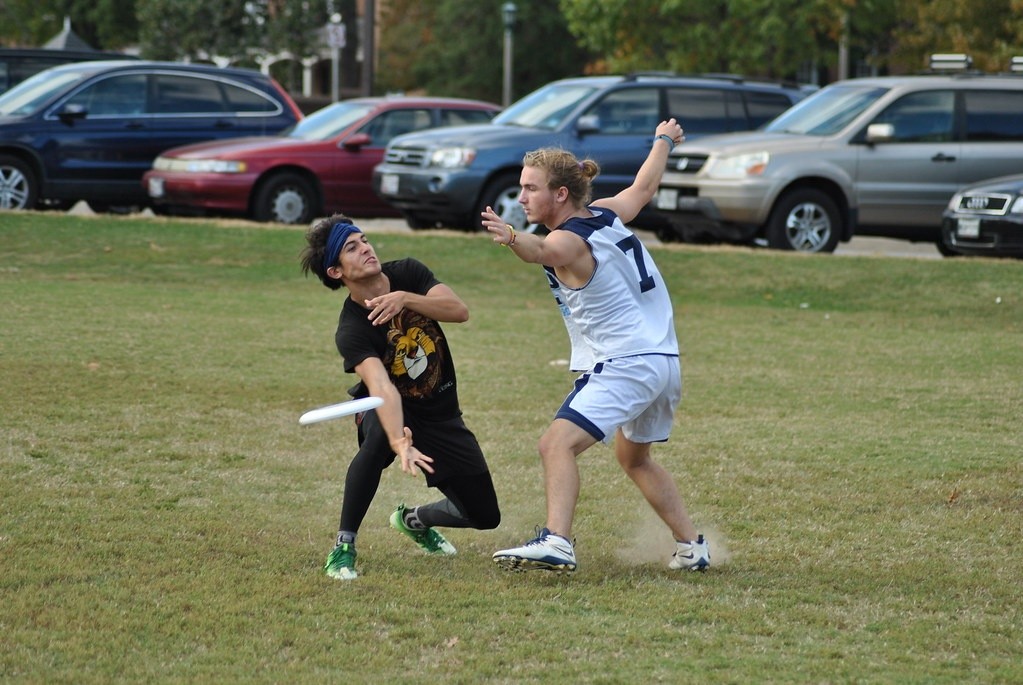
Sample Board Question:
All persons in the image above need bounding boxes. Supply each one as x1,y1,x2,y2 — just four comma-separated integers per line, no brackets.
481,118,711,574
303,216,500,580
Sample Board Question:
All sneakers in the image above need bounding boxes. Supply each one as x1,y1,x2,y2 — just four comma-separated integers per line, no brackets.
492,525,578,576
669,534,712,573
389,503,457,557
323,544,357,580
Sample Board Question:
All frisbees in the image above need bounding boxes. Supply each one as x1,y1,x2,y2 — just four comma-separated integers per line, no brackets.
297,395,385,426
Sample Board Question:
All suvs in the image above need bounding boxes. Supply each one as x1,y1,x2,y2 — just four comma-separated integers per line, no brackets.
0,49,153,110
641,70,1023,259
0,60,306,212
372,71,823,235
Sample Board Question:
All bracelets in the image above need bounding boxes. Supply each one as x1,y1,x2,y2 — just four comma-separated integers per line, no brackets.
500,224,516,247
653,134,681,152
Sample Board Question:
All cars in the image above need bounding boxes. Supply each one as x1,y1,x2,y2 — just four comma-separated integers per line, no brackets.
939,172,1023,260
142,92,508,226
